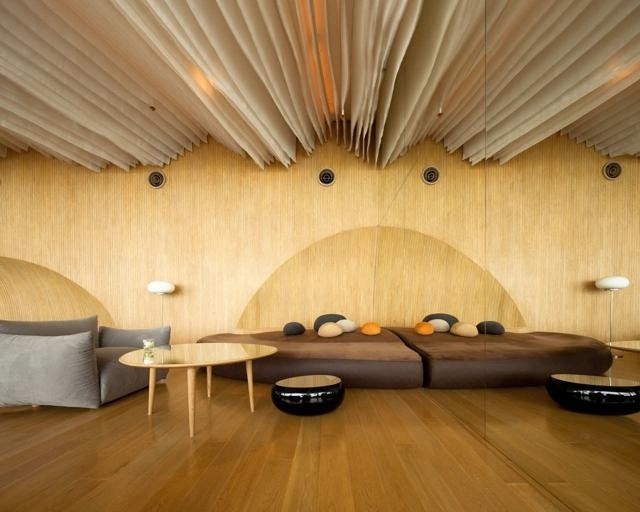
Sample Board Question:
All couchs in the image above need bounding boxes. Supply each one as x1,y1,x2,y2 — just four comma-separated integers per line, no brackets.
0,315,171,408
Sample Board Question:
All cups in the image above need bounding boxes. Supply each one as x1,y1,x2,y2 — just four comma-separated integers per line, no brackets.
143,352,154,364
143,339,155,352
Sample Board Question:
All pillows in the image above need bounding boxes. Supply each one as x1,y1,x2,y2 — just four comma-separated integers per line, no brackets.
283,314,381,336
415,313,505,337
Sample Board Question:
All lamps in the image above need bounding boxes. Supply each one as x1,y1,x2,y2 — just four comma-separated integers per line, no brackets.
148,281,175,328
595,276,629,357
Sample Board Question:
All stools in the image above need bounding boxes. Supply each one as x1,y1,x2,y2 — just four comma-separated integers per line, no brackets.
545,370,640,416
271,375,345,416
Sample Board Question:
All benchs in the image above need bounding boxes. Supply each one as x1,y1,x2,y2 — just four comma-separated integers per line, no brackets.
386,327,613,389
196,325,424,390
